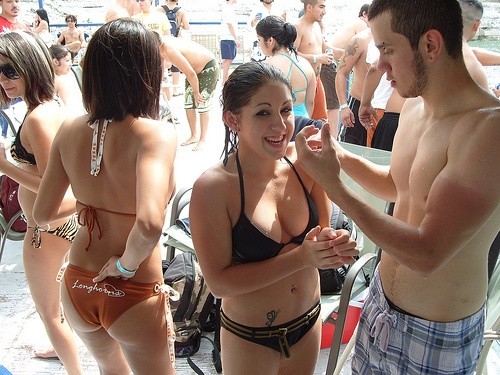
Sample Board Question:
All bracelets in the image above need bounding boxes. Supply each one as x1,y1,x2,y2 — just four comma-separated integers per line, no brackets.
116,259,136,275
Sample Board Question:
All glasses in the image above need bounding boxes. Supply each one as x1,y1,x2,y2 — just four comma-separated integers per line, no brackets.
0,63,21,80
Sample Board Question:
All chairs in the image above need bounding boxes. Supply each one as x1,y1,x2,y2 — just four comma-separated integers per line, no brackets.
0,207,28,263
326,200,500,375
166,185,357,263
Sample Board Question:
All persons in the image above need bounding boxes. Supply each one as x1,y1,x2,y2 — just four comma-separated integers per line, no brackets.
0,30,84,375
293,0,500,152
295,0,500,374
189,62,359,375
256,16,317,118
106,0,189,103
32,19,176,375
0,0,86,116
152,30,219,151
248,0,280,63
219,0,240,85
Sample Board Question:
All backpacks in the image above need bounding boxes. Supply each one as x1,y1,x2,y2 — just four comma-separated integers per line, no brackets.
161,5,182,37
162,253,217,359
0,175,28,232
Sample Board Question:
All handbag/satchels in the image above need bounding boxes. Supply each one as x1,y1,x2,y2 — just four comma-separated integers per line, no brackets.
318,268,342,294
